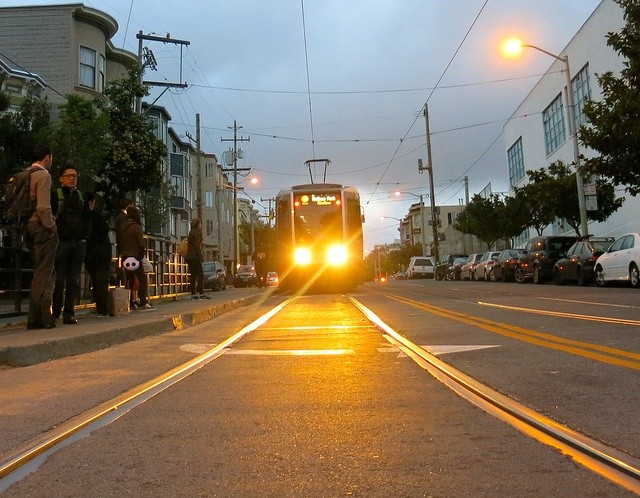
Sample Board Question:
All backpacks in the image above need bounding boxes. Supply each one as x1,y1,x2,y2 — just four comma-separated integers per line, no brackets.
4,166,44,234
56,187,89,241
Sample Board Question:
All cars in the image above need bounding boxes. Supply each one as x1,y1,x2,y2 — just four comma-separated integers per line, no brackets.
593,233,640,287
553,241,615,287
266,272,278,285
449,257,467,280
490,249,523,281
462,253,483,279
395,273,404,279
200,261,226,291
473,252,502,281
234,265,260,288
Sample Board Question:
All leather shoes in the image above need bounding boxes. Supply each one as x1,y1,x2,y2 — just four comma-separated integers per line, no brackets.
63,318,77,324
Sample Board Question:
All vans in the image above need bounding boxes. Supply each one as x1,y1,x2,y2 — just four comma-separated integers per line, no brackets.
515,236,577,284
436,254,469,281
407,257,434,279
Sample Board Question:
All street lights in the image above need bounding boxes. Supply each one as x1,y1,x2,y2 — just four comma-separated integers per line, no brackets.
381,216,406,275
395,191,427,257
236,177,258,186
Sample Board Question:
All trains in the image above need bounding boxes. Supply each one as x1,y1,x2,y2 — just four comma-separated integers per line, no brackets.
277,184,366,293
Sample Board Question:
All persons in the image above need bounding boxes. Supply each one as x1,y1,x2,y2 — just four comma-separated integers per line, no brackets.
176,219,211,301
5,147,60,330
119,207,154,312
83,192,116,318
251,242,271,288
114,200,134,289
50,164,90,324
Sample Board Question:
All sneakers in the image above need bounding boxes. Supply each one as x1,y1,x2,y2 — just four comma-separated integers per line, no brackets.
200,294,211,299
131,302,140,309
191,295,203,301
139,303,157,311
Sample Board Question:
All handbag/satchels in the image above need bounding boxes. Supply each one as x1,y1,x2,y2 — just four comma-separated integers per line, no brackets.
177,237,189,257
